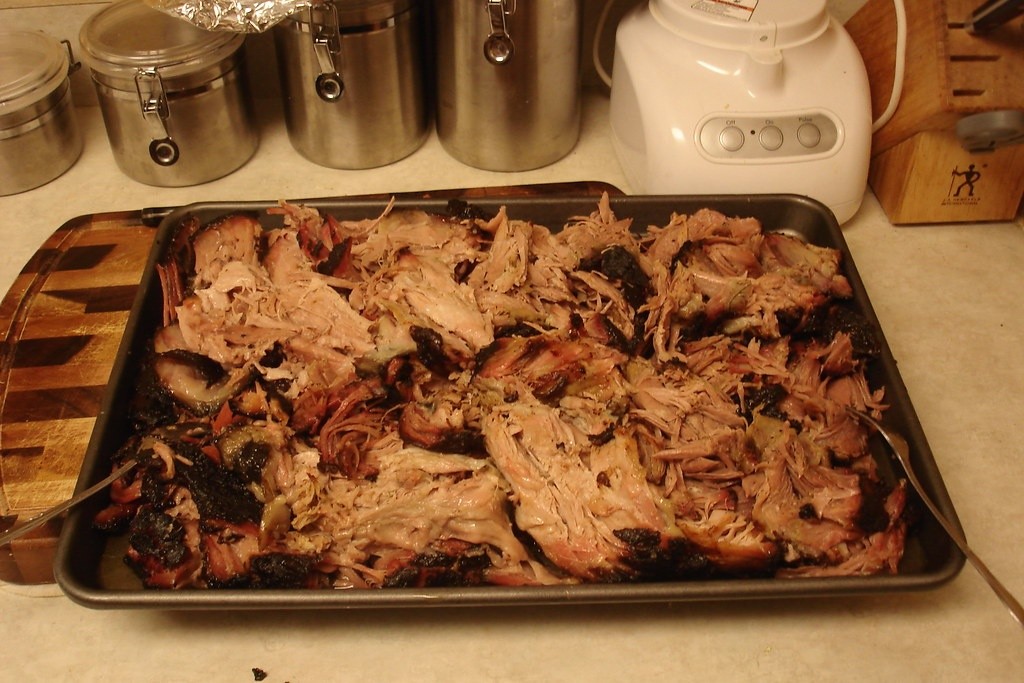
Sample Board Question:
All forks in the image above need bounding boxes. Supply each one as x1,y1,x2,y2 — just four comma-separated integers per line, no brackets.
849,408,1023,627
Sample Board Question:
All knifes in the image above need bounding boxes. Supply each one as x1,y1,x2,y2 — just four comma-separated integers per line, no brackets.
965,0,1024,35
